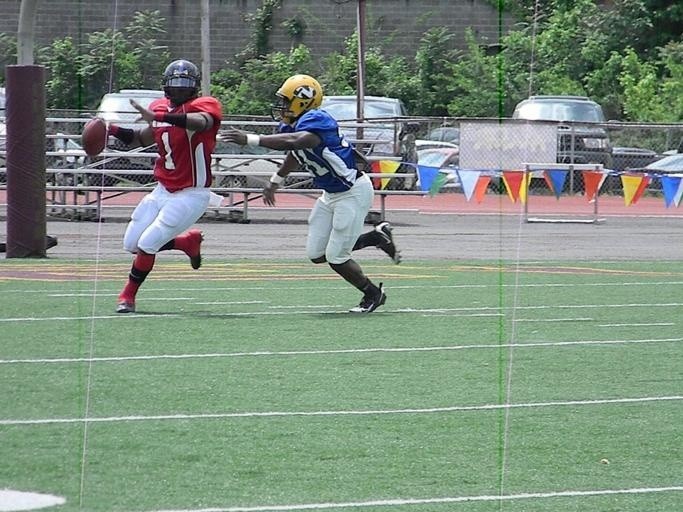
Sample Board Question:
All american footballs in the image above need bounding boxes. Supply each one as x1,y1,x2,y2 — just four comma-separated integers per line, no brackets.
82,118,108,155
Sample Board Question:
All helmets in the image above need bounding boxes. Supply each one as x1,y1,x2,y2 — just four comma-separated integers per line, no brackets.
270,74,324,127
160,59,201,106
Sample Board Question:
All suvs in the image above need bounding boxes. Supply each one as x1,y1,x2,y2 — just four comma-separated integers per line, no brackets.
513,95,610,191
89,90,165,185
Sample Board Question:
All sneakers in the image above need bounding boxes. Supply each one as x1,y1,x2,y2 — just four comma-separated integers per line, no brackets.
116,289,136,313
349,282,386,313
373,220,402,265
187,230,206,270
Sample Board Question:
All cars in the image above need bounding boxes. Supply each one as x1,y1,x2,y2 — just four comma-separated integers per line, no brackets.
322,95,460,192
613,146,663,189
209,133,283,186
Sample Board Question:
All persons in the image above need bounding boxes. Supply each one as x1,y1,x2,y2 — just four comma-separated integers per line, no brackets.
104,58,222,313
219,73,403,315
